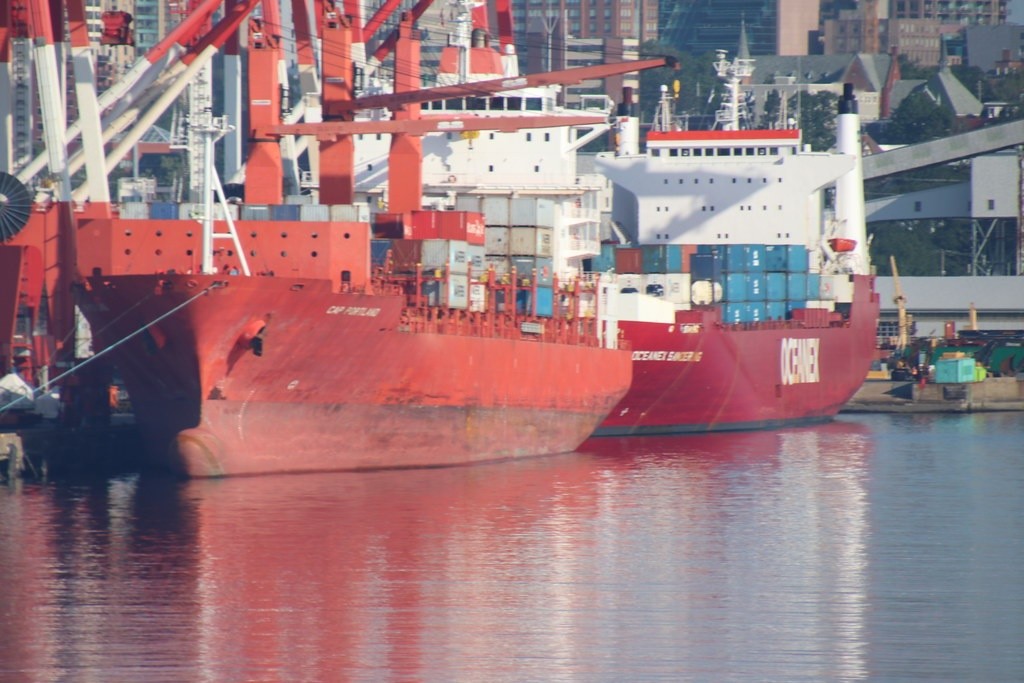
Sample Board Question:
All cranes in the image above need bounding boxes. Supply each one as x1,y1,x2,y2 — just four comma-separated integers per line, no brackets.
890,255,912,351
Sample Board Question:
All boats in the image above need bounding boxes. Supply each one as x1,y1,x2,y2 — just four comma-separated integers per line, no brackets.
67,259,636,482
543,222,883,440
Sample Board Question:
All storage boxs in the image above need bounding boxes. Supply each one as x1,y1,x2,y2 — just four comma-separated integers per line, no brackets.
120,194,821,325
935,353,975,383
